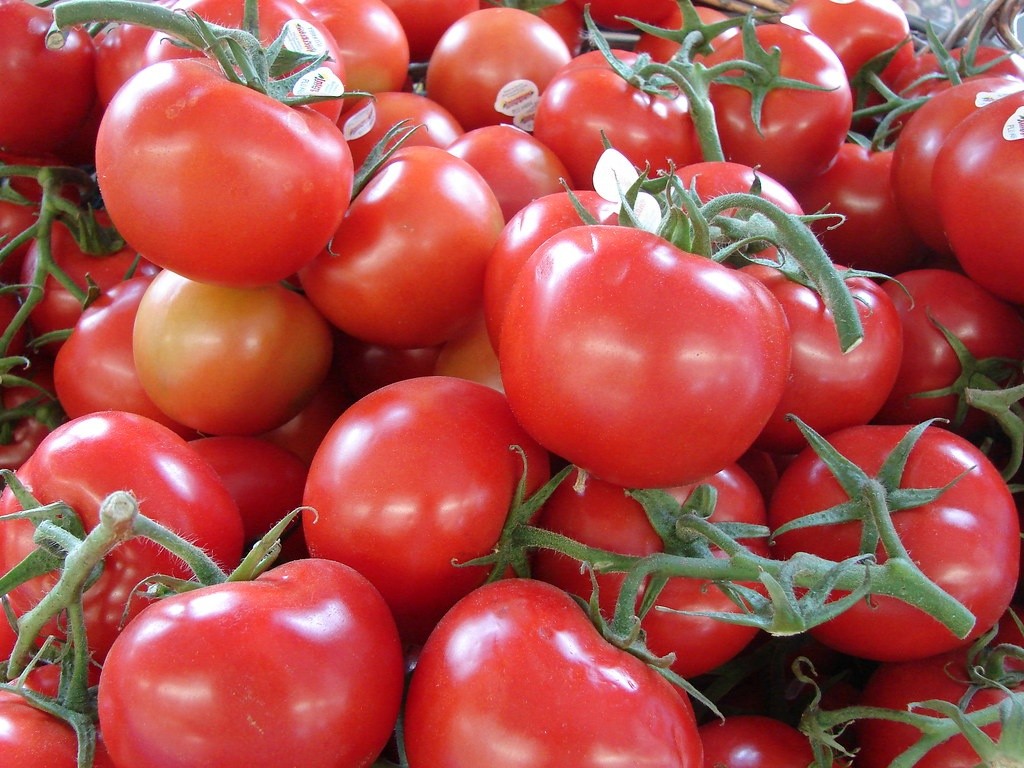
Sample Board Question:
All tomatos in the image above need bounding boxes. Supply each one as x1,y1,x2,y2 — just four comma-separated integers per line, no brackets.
0,0,1024,768
